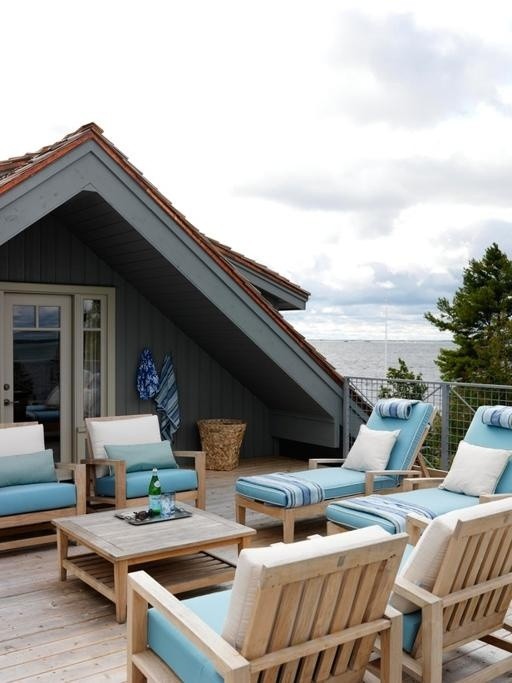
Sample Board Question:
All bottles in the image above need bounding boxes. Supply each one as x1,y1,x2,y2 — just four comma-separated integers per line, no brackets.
148,465,162,514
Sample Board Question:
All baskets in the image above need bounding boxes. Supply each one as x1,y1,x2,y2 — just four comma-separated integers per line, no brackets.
197,419,247,470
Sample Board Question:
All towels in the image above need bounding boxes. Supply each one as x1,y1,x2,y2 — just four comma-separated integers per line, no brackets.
136,348,159,399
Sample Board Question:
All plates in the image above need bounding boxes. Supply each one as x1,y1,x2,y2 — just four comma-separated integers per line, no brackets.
114,506,193,526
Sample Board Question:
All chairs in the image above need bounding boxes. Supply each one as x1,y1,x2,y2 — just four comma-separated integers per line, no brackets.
124,524,402,683
80,413,207,516
324,404,512,537
1,421,85,555
235,398,438,553
306,497,512,683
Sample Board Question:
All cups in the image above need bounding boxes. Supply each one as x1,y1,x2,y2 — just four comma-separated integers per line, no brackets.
160,493,171,519
166,490,176,515
